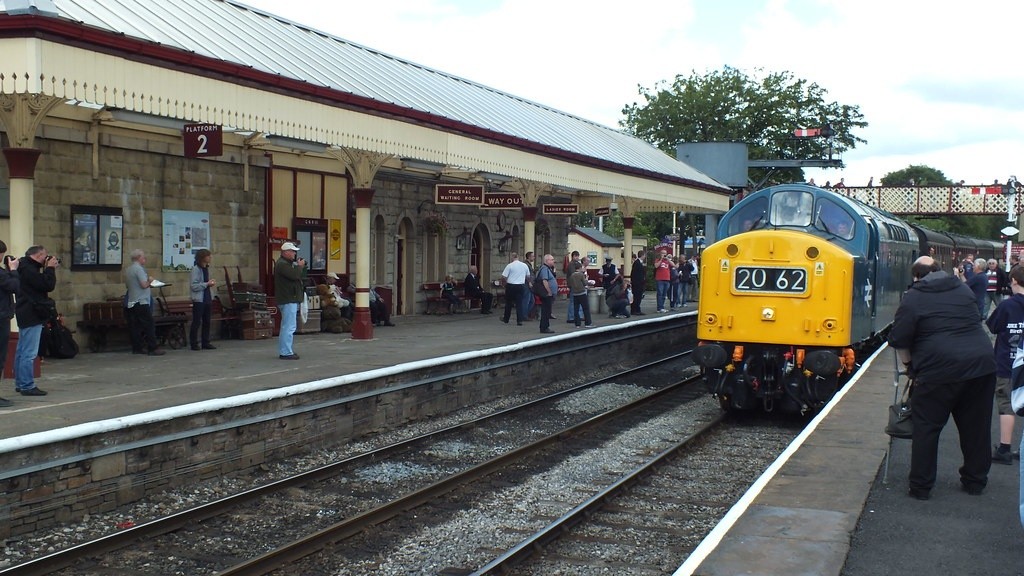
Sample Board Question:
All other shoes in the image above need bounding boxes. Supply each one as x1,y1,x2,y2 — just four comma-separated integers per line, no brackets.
191,346,200,349
677,304,681,307
203,344,217,349
683,304,687,307
657,309,669,313
0,397,14,406
149,350,166,355
384,321,396,326
16,387,21,392
21,387,48,395
279,354,300,360
990,447,1013,464
499,314,596,333
686,299,699,302
481,310,489,314
132,349,147,354
488,310,492,313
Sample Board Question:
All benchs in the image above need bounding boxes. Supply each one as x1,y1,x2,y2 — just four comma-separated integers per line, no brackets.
76,296,237,351
422,282,480,314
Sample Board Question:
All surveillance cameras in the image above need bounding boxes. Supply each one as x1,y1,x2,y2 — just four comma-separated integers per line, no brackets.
609,202,618,212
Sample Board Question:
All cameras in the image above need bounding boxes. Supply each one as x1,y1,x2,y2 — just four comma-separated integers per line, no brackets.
298,256,308,265
44,256,61,264
3,255,15,265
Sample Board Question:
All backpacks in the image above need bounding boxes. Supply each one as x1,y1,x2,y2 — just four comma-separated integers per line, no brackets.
47,324,78,359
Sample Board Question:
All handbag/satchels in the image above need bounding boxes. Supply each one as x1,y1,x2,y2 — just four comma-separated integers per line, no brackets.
33,298,58,321
530,268,550,300
884,378,913,439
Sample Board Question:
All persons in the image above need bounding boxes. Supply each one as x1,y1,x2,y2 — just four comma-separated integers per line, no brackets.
464,265,493,314
598,250,648,319
566,251,596,328
440,275,467,315
274,242,308,360
123,249,166,356
826,219,850,238
327,272,353,321
807,177,1011,188
369,286,396,327
985,265,1024,526
500,252,558,333
14,245,60,396
929,246,1024,324
743,213,764,232
654,250,699,313
887,255,997,500
189,249,217,350
0,240,19,407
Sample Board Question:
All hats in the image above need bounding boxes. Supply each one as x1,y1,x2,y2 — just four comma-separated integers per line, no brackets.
281,242,300,251
328,272,340,279
605,257,613,260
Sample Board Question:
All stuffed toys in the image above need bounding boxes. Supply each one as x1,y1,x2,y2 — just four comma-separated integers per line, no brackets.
317,284,352,334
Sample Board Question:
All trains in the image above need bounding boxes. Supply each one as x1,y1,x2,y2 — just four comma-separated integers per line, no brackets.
693,183,1007,422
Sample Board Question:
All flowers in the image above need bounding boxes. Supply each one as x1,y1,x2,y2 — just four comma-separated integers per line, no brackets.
424,204,450,235
